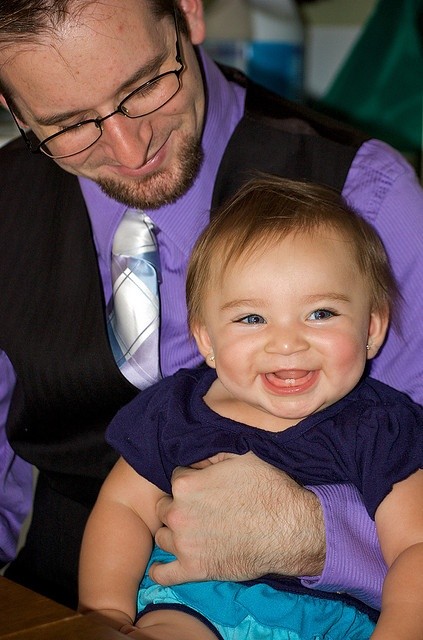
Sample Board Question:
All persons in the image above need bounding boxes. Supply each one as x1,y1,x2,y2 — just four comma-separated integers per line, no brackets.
1,0,422,615
77,171,422,640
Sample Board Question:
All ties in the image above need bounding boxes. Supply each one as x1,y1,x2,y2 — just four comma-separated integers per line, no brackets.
106,209,162,394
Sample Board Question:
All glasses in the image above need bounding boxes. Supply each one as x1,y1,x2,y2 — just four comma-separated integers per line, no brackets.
5,3,186,160
